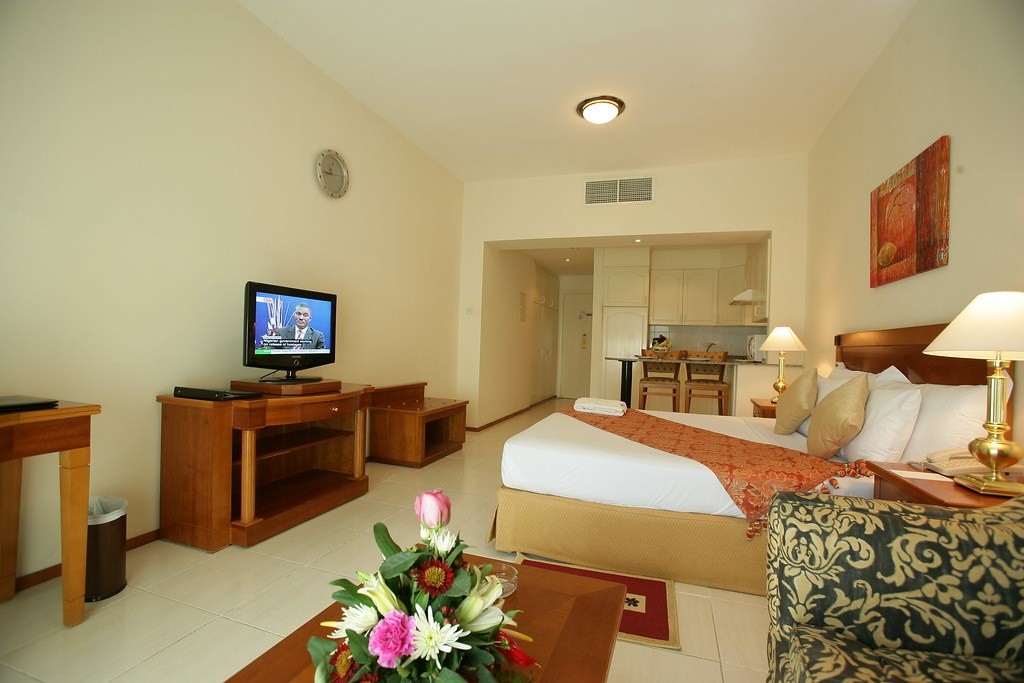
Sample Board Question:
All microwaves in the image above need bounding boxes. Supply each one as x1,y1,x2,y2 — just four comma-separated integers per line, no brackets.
747,335,766,362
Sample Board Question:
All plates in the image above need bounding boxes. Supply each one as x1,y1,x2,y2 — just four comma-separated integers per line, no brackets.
639,357,654,359
734,359,753,363
651,348,671,360
686,358,711,361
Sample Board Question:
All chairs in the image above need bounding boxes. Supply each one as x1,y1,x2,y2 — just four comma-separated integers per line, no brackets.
639,349,683,413
684,351,729,415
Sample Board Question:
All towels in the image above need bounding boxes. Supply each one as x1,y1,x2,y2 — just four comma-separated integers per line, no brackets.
573,398,627,416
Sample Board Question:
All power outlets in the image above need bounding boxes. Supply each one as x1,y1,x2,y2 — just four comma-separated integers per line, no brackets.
698,341,717,351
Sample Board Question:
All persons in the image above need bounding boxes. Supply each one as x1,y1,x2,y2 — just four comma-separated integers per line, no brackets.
274,302,325,349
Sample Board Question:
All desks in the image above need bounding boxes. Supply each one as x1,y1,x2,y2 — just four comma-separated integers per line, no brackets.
0,398,102,627
602,355,753,412
225,542,627,683
368,398,469,469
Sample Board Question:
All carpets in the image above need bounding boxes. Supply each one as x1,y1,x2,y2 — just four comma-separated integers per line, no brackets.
509,550,681,653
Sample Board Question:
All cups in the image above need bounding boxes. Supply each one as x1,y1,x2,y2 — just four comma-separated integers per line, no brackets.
678,350,688,360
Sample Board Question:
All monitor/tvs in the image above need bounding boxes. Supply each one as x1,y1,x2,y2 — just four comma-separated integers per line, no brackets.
243,280,337,384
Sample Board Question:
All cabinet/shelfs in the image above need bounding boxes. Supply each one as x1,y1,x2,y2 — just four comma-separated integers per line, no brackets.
650,239,770,326
155,375,378,555
530,264,559,403
602,266,649,410
646,365,803,416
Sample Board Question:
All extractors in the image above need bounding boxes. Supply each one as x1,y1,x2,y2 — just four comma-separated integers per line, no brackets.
729,289,752,305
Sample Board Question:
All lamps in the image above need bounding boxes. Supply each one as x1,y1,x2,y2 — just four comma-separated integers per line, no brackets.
575,93,627,126
922,289,1024,501
758,325,808,402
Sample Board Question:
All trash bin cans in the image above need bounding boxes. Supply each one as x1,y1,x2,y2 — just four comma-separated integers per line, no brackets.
84,495,129,603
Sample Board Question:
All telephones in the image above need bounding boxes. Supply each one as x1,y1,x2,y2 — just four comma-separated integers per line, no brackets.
923,447,1024,477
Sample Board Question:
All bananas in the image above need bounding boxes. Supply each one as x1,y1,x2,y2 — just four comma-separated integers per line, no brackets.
658,337,670,346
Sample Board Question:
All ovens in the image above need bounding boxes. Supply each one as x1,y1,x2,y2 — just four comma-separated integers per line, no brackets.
750,304,762,321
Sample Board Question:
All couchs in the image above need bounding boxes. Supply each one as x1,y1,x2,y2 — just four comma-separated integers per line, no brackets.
765,489,1024,683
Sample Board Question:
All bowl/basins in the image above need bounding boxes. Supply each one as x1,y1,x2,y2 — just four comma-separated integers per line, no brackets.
482,563,518,599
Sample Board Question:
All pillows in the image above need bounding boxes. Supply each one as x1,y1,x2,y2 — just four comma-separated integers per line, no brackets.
743,361,1013,467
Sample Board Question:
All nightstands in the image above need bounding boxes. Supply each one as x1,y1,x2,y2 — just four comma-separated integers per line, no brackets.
864,458,1024,521
749,395,795,418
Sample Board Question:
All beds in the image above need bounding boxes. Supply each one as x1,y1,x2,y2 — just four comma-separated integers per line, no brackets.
488,323,1024,594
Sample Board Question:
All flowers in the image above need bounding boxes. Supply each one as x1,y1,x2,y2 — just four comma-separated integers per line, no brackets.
306,489,539,683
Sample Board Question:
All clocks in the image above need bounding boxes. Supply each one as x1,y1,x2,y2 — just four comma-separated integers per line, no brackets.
315,149,352,200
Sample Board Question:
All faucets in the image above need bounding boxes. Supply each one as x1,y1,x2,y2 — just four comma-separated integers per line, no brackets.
706,343,718,352
